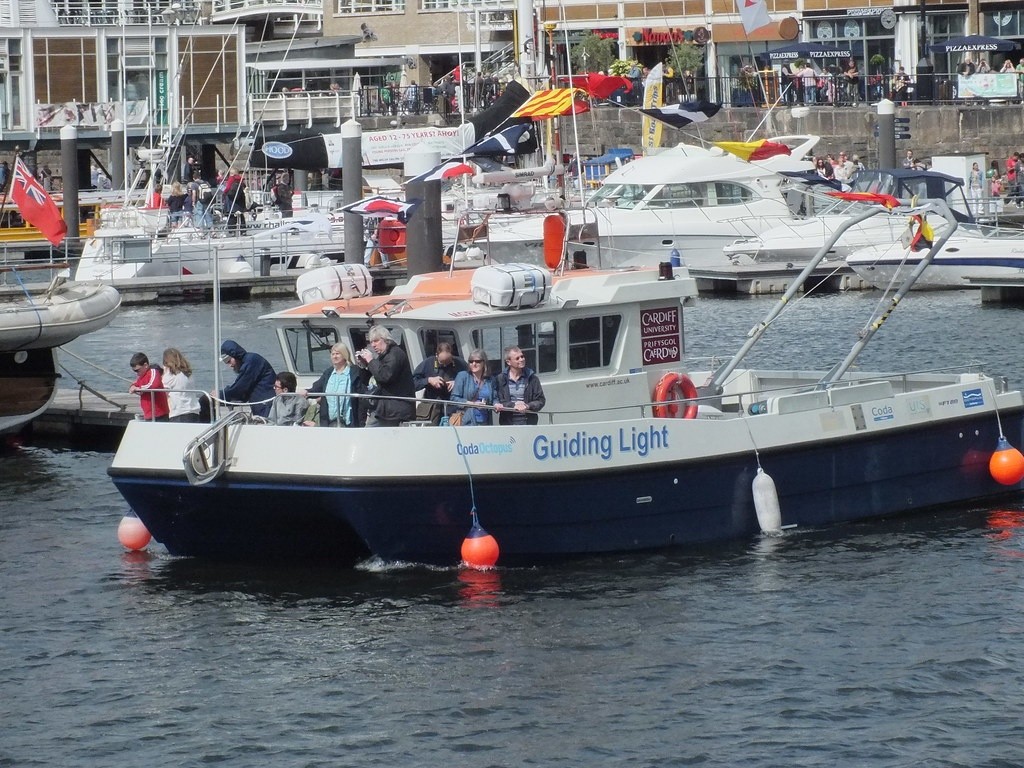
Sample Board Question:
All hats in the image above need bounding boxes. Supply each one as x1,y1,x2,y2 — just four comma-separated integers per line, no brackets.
218,353,230,364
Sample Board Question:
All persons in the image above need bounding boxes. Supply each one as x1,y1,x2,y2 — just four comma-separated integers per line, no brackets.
783,59,911,107
128,325,545,427
970,152,1024,214
278,73,499,113
0,161,10,190
629,63,694,104
148,158,247,236
903,151,931,170
90,166,102,187
956,57,1024,106
816,152,864,189
276,174,293,219
40,165,52,180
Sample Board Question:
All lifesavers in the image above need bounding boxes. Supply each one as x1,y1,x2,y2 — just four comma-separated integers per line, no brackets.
653,372,699,419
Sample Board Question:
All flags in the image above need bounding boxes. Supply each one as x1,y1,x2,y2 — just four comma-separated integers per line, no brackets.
827,190,933,253
341,162,473,222
713,139,792,163
736,0,772,33
641,100,724,129
576,73,632,98
37,102,115,125
9,154,68,247
463,88,588,157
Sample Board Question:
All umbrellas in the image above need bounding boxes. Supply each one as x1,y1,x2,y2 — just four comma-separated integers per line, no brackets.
759,42,852,59
929,34,1021,65
353,72,362,95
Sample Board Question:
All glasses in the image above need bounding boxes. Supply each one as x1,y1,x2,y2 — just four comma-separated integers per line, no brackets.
273,384,284,389
133,366,144,372
468,359,483,364
510,354,525,361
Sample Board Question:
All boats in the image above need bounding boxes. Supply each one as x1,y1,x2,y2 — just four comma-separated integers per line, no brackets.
1,1,1023,295
0,235,155,442
108,211,1023,565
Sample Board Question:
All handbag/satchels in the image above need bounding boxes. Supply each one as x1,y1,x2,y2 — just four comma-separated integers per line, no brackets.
416,401,441,427
448,411,464,426
361,387,382,408
